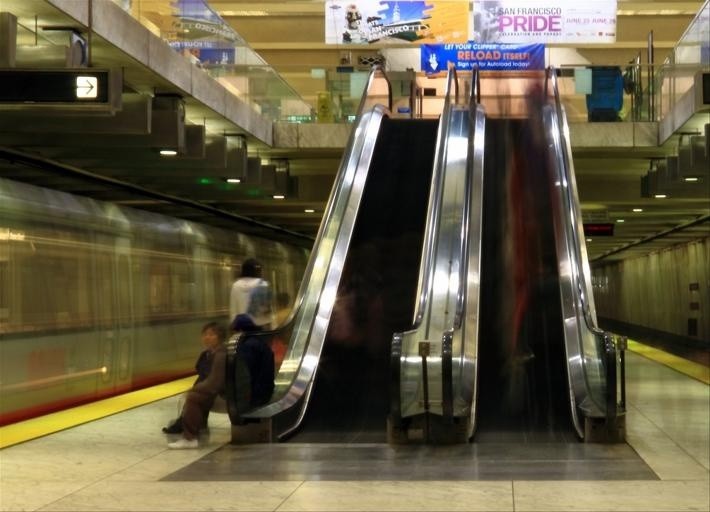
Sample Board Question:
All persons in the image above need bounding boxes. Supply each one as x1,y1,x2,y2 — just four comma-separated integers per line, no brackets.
167,314,274,450
229,258,277,345
270,293,293,370
345,5,362,30
162,322,225,433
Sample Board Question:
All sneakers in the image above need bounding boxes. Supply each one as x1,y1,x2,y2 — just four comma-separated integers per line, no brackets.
162,422,183,435
167,437,199,450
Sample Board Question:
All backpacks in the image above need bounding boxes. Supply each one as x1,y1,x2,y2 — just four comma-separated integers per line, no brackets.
247,278,274,317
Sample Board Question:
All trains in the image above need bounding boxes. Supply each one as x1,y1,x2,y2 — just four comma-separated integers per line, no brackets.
0,176,312,430
376,17,434,36
